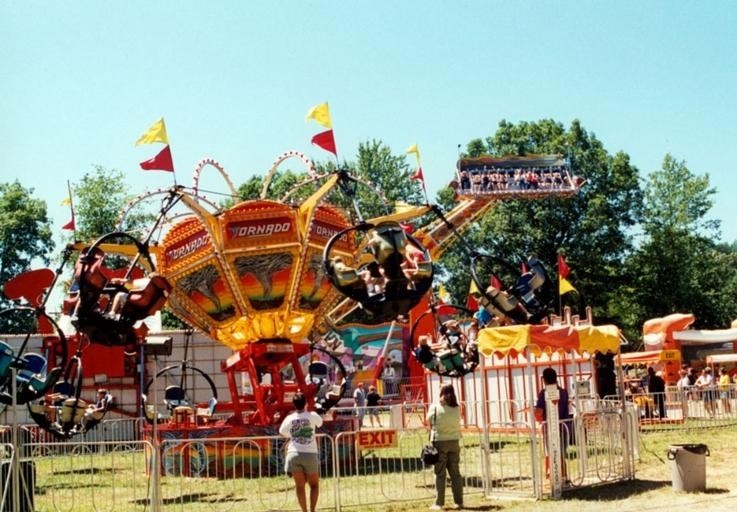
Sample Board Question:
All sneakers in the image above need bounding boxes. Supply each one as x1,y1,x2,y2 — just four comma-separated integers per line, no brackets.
430,504,442,510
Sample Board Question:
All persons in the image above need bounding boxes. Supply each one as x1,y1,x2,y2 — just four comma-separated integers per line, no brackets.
98,270,161,323
422,381,469,510
352,382,366,430
459,167,571,191
364,384,383,427
315,233,531,407
533,366,571,488
69,387,108,434
277,390,325,511
628,362,736,419
26,373,65,431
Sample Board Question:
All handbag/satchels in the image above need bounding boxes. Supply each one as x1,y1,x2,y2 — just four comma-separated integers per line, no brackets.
421,445,440,465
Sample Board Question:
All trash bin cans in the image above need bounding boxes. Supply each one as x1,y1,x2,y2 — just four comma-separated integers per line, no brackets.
667,444,711,492
